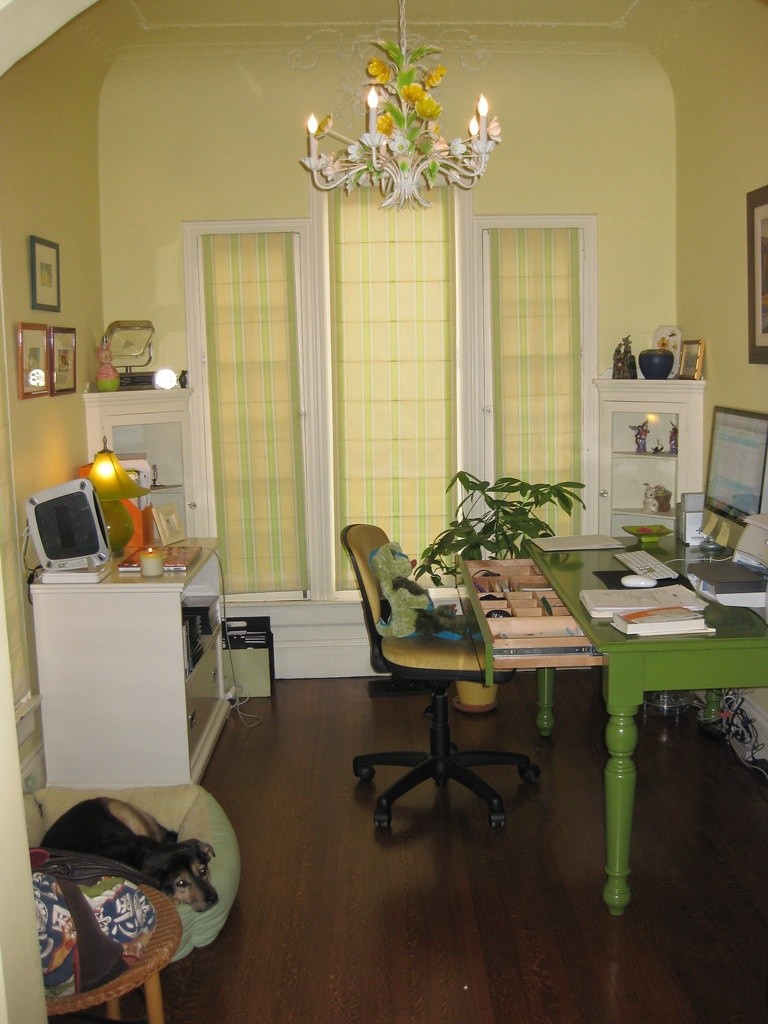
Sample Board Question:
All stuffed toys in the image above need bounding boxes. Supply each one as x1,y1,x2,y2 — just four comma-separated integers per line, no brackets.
369,541,465,637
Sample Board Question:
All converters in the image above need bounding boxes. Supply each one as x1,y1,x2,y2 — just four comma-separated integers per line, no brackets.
698,724,727,742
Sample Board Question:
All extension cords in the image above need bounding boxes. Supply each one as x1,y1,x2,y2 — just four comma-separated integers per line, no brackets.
732,734,752,762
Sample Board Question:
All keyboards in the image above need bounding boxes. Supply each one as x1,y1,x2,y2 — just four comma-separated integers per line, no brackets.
613,549,679,580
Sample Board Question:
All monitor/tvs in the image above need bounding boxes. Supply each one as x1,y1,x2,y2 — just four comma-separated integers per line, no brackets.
700,405,768,560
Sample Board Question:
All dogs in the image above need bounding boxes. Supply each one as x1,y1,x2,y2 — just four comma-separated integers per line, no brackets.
38,797,220,911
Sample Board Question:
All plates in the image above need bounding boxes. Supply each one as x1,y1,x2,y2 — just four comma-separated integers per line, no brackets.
621,524,673,542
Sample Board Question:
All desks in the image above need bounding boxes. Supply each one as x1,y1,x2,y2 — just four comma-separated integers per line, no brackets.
41,880,183,1024
454,540,767,912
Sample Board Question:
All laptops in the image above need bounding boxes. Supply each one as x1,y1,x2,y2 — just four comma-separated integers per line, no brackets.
117,545,203,571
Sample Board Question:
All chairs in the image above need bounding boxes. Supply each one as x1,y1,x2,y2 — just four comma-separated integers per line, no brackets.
340,519,547,831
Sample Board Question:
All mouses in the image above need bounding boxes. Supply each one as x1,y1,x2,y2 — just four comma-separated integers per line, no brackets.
621,575,658,587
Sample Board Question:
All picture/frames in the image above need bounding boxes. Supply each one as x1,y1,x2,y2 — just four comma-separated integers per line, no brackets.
677,339,704,380
745,185,768,365
30,234,61,310
18,322,49,399
152,504,188,544
51,326,77,397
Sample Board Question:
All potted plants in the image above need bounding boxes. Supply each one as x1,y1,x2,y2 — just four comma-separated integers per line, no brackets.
416,473,583,711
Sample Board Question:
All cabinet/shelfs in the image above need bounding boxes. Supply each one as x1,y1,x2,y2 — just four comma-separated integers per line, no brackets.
30,536,234,786
593,377,702,538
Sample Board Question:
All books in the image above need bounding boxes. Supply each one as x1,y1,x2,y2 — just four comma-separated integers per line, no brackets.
610,606,716,636
181,594,269,678
118,544,202,571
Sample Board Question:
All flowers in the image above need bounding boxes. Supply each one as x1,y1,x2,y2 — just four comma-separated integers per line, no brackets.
644,331,676,358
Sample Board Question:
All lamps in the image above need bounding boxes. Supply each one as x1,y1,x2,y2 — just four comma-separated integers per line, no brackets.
89,437,150,559
297,0,503,210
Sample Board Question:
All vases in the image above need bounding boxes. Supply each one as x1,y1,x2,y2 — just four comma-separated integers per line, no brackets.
640,349,674,380
